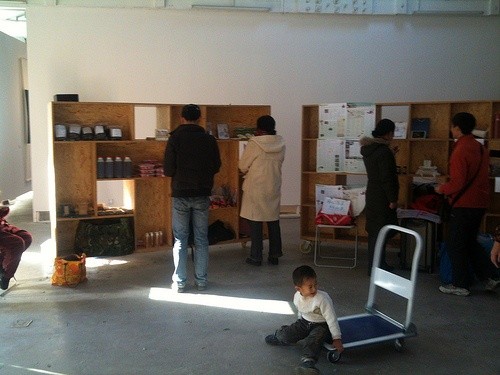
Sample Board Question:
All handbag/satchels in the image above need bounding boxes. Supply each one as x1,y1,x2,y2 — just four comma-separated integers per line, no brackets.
51,252,87,287
440,202,452,222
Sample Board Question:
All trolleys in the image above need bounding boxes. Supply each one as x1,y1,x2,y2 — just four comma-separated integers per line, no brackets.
322,224,423,365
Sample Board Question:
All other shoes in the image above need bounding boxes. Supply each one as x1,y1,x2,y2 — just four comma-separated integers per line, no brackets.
192,280,207,291
368,263,393,276
300,356,316,368
0,264,10,290
267,257,279,265
265,334,284,345
246,256,262,267
171,283,184,292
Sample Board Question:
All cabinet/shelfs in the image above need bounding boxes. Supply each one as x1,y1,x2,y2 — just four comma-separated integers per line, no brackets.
47,100,271,259
300,100,500,255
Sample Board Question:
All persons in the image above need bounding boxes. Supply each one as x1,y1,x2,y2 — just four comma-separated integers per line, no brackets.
265,265,344,375
238,116,286,266
430,111,500,296
358,117,399,276
165,104,222,293
0,205,33,291
490,224,500,269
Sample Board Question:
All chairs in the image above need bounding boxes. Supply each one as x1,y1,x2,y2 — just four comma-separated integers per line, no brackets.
314,186,358,269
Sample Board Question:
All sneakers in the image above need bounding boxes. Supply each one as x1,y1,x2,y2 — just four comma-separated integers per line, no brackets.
438,282,473,296
483,276,500,291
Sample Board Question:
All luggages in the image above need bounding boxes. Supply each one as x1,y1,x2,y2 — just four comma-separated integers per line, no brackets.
397,217,444,274
